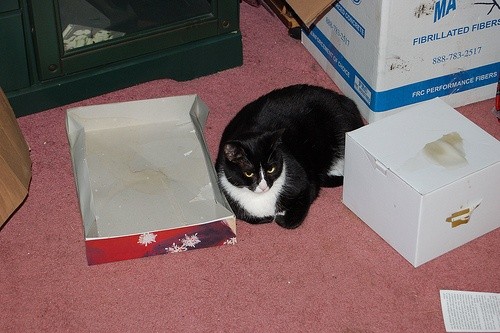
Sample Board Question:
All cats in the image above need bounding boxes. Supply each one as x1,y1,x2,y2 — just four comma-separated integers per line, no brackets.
214,84,366,229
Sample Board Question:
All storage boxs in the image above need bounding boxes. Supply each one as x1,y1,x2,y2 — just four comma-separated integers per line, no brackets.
65,93,237,266
342,96,500,267
300,0,499,126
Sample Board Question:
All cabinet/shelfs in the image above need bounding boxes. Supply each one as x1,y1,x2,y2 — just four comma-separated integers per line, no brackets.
0,0,243,119
